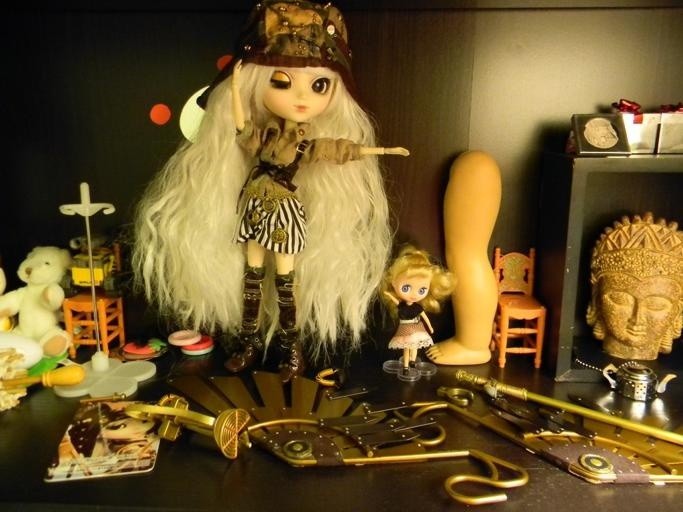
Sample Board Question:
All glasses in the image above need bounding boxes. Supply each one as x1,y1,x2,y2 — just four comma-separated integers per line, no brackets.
69,404,110,452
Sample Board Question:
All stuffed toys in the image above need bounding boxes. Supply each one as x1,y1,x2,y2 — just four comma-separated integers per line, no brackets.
0,246,72,358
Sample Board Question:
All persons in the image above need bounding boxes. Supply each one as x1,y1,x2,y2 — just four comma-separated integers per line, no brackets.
80,401,160,471
132,1,411,379
587,211,682,362
379,247,455,374
